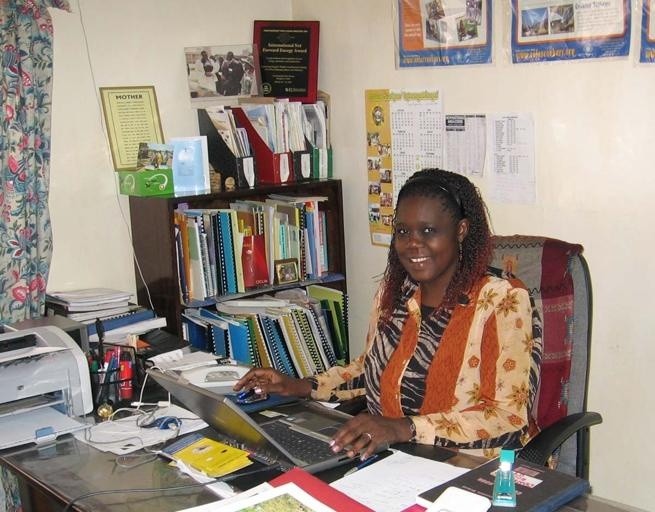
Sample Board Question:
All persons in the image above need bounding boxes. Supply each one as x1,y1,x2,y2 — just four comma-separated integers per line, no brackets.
136,145,173,168
426,0,481,41
232,164,543,461
186,50,255,98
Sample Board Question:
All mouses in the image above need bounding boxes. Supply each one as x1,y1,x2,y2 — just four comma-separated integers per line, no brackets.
236,386,270,404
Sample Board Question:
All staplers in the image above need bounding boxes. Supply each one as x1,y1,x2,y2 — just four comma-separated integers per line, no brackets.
492,450,517,508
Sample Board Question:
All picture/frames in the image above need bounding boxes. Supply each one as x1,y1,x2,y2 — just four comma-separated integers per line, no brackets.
253,20,320,104
100,86,165,172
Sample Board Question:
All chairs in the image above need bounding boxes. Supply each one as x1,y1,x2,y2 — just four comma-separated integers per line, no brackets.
332,235,602,480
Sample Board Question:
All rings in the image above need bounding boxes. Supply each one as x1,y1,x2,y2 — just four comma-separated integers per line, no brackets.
362,431,376,441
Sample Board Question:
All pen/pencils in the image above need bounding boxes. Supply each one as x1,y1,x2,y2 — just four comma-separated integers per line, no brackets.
343,454,379,476
84,345,120,405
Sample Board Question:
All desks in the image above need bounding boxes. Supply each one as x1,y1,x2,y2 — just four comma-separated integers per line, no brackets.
0,395,638,512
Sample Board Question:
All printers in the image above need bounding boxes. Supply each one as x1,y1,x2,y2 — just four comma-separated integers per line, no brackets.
0,325,93,452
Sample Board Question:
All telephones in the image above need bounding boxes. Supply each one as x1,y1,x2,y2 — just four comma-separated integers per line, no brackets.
179,364,251,396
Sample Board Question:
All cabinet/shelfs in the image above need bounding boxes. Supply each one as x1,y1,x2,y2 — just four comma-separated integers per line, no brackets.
130,179,349,381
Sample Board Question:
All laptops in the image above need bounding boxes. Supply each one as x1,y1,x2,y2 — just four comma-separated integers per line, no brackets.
145,368,372,474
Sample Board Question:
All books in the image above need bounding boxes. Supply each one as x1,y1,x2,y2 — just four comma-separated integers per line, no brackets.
45,284,134,312
172,192,349,380
